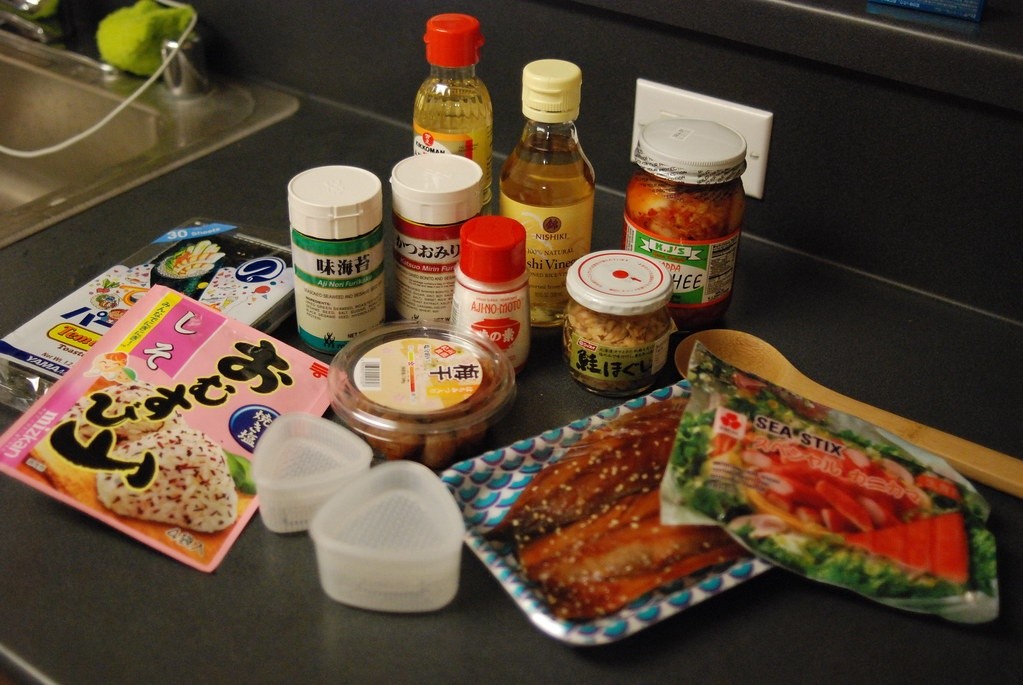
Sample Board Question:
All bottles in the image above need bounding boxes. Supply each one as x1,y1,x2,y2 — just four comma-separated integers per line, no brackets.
391,154,487,325
455,216,530,377
284,164,384,356
493,59,596,334
410,12,492,220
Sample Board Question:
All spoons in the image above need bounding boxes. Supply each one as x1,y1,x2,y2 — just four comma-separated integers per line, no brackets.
672,329,1023,501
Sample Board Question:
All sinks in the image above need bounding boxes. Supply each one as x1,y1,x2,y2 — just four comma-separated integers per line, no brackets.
0,27,302,252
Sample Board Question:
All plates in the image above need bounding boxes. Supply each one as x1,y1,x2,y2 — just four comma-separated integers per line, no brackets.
436,381,776,648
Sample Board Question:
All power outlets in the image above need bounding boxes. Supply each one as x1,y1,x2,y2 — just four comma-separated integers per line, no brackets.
629,78,774,199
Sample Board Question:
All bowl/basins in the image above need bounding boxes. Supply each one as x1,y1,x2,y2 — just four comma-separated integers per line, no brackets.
328,322,517,469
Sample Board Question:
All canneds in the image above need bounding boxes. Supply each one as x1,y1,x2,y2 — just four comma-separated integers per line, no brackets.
622,117,747,332
563,250,673,398
285,164,384,355
390,154,484,326
